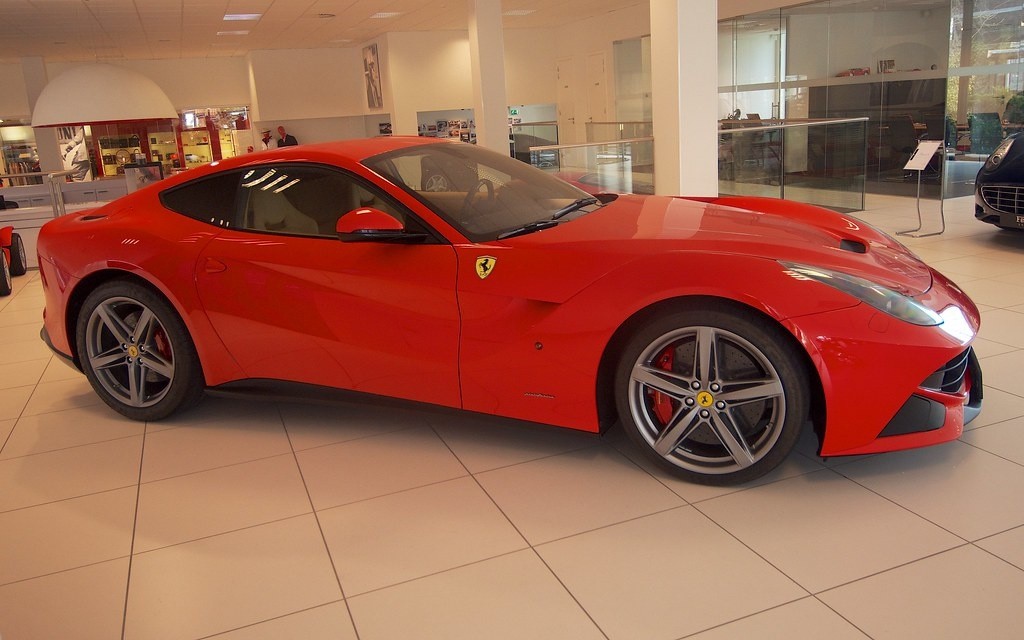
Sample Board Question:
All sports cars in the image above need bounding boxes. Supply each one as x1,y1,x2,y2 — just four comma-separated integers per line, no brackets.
37,134,984,487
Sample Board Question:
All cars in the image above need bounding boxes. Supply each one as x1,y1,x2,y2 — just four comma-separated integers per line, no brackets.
974,130,1024,232
0,226,28,296
471,133,558,165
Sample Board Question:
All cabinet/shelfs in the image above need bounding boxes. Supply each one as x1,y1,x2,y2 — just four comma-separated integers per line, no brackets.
83,113,250,180
2,141,39,163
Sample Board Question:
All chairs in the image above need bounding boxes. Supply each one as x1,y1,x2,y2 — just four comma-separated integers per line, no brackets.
251,183,319,235
743,113,781,168
888,114,1002,185
349,161,406,228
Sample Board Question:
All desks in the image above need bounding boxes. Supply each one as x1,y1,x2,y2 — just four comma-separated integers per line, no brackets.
955,123,1024,130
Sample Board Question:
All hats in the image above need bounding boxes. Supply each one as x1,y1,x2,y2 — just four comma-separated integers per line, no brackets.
259,128,271,134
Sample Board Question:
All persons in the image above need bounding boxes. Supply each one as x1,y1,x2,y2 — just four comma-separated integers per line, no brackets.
260,128,278,150
278,126,298,146
